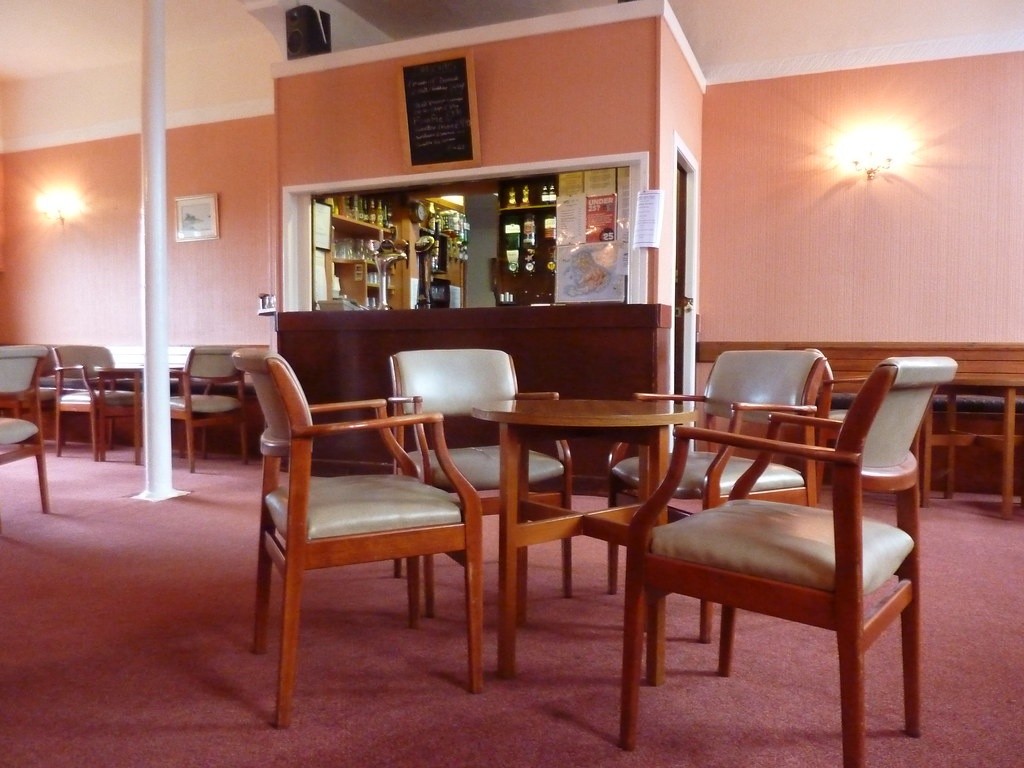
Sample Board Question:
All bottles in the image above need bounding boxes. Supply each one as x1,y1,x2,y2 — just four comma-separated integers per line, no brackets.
436,208,441,232
541,186,549,204
376,200,383,227
432,222,440,256
363,198,368,223
359,197,364,221
369,199,376,224
427,202,436,230
549,186,556,204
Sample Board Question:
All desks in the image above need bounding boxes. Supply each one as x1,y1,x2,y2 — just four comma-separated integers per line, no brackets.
471,398,700,687
92,366,188,464
921,376,1024,519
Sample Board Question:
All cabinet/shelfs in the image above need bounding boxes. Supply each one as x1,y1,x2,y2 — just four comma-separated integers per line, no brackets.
331,212,397,304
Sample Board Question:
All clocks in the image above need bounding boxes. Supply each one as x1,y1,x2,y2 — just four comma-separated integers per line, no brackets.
409,201,428,224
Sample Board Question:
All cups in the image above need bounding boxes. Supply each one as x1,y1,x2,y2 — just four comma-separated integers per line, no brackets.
368,272,390,286
367,297,378,307
259,297,269,309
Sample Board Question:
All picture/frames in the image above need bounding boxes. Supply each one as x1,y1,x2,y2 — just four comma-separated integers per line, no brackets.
173,192,222,243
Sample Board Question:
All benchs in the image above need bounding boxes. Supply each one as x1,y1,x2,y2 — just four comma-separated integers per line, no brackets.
825,390,1022,496
38,375,262,454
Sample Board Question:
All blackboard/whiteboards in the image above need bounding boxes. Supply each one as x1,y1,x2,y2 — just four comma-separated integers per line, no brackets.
395,47,482,173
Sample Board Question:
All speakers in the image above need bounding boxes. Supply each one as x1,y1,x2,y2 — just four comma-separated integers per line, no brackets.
286,5,331,60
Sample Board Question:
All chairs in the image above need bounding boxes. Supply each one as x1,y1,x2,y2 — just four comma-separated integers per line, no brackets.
169,344,249,473
232,347,486,731
641,354,960,767
0,345,49,534
389,348,574,618
805,346,869,505
53,345,144,462
605,348,834,645
